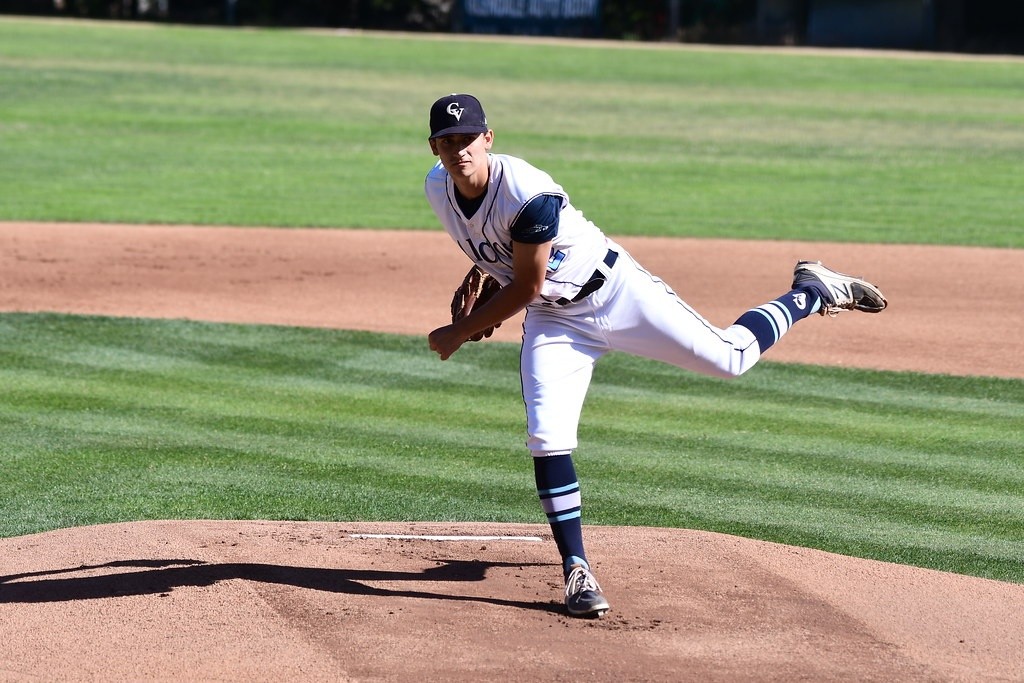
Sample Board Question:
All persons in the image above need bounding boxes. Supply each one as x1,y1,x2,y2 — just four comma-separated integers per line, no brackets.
424,94,888,619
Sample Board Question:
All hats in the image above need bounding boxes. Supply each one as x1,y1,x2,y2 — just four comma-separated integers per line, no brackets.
430,93,488,139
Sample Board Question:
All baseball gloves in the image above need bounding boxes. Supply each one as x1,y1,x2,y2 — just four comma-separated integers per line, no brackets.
451,267,504,342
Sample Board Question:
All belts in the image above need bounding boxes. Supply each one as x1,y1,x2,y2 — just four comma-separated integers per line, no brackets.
542,249,618,305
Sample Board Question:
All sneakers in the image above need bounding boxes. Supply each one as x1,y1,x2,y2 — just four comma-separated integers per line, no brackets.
792,259,888,318
563,553,609,615
